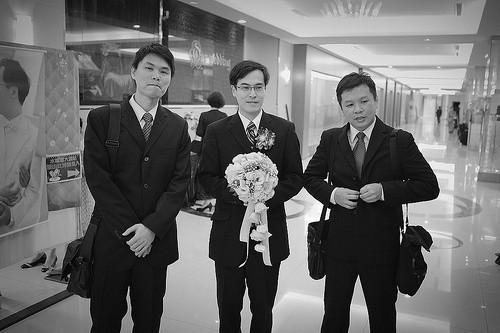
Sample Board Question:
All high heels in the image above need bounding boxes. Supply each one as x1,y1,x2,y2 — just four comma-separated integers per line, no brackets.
41,255,58,272
196,202,212,212
21,253,47,269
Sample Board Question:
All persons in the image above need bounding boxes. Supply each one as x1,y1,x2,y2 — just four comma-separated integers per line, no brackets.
196,91,228,212
303,72,440,333
437,106,442,123
448,106,457,134
84,44,191,333
0,59,45,236
198,61,304,333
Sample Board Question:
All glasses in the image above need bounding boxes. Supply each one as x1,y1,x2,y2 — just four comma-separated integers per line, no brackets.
234,85,265,92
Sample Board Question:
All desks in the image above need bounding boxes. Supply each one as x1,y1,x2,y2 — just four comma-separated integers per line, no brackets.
182,152,203,208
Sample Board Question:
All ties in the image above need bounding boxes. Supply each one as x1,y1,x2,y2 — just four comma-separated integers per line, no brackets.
143,112,153,143
246,122,257,150
353,131,367,179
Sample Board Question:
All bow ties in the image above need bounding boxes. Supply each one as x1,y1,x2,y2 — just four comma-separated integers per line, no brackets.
0,115,20,131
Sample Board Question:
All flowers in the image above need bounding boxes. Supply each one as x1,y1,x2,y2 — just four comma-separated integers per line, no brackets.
254,127,276,152
224,153,278,267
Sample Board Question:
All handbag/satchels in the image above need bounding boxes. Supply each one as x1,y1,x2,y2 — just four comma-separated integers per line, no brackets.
396,225,433,297
61,224,96,299
190,134,202,155
308,220,328,280
